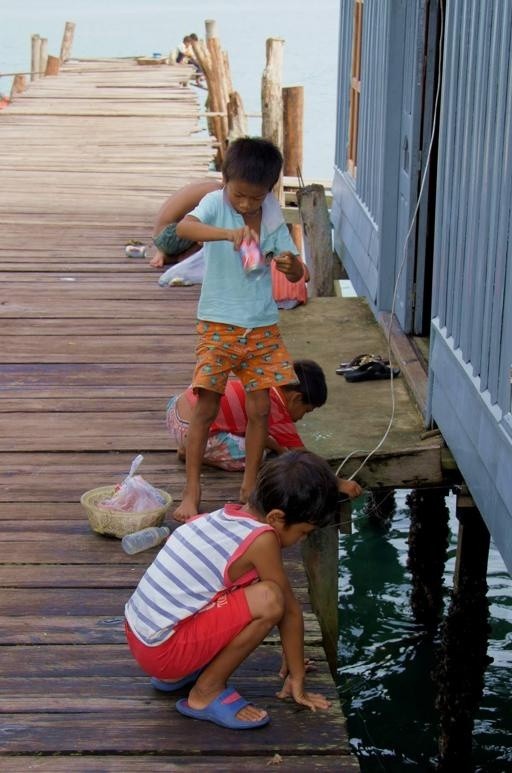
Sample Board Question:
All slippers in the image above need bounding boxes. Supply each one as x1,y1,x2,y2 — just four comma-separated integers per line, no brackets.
151,664,203,691
174,687,270,729
335,353,401,382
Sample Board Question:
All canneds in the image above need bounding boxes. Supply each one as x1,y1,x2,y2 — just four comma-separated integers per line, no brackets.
238,234,264,276
124,244,147,258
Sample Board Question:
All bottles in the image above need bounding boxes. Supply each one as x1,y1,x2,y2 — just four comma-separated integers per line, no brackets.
121,525,170,556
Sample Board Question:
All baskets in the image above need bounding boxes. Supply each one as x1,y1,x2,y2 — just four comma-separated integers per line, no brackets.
81,486,172,538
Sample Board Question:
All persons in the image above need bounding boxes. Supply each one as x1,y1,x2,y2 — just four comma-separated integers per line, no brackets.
172,136,304,522
150,180,226,267
167,357,363,499
124,447,333,732
